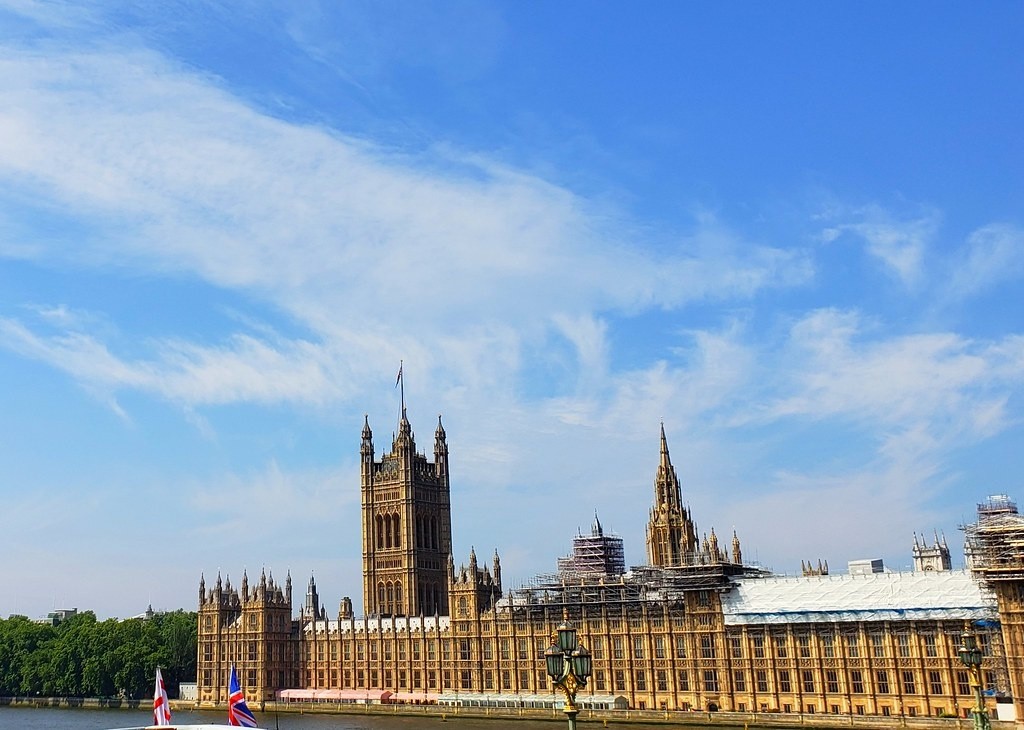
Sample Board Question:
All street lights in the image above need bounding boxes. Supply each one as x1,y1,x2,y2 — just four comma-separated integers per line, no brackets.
543,606,594,730
959,624,990,730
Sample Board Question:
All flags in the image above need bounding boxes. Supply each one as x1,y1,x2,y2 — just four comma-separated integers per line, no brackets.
154,669,170,725
228,665,258,728
